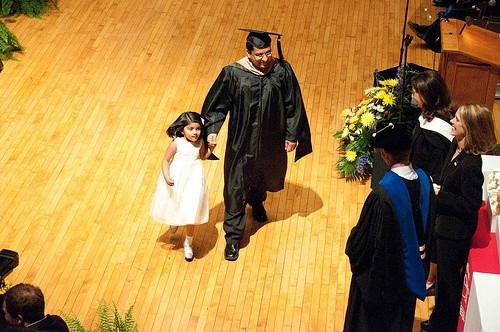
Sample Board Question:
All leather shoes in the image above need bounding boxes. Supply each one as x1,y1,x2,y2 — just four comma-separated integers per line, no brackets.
224,242,239,260
249,201,267,222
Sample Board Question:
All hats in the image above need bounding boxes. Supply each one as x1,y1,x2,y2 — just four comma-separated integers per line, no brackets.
369,118,412,153
238,29,284,61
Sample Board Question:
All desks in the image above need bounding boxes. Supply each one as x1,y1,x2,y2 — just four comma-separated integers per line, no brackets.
457,155,500,332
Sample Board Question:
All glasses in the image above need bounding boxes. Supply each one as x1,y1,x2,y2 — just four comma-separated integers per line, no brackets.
249,51,272,59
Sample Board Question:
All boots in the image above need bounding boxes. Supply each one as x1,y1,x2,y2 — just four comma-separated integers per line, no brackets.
407,15,440,46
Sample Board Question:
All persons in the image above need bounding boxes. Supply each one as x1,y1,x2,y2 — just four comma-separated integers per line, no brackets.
343,0,500,332
201,28,312,261
150,112,217,259
0,283,70,332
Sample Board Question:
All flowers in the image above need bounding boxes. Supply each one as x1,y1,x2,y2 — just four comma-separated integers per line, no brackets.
334,78,399,180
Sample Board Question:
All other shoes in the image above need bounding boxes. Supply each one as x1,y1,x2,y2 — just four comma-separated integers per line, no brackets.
183,242,193,259
425,283,436,296
169,225,178,234
